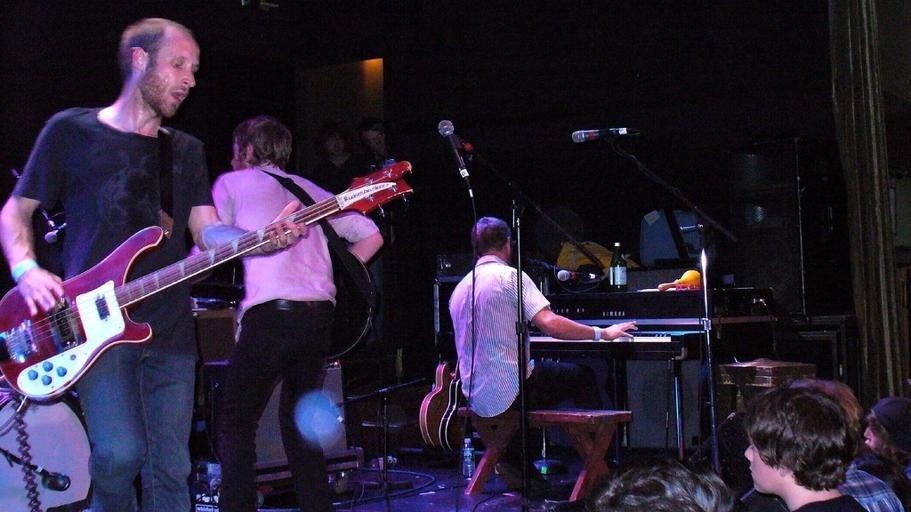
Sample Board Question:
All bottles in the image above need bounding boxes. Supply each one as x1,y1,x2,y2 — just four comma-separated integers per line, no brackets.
462,438,475,476
609,242,628,292
369,456,397,470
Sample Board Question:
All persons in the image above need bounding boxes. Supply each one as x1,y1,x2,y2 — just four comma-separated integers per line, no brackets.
0,16,306,510
212,114,384,512
361,120,390,174
865,397,911,512
742,386,868,512
309,128,361,193
738,377,911,512
595,456,744,512
449,214,638,493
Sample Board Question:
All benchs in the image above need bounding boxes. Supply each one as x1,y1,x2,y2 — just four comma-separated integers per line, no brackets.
455,406,632,503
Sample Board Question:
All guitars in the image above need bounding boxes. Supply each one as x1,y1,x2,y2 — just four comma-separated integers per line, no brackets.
190,242,380,362
419,358,456,454
0,156,414,402
438,357,474,459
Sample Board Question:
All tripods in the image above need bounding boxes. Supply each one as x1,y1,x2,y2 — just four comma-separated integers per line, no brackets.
362,394,412,500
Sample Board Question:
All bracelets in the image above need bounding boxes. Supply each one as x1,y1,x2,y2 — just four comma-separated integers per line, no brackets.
11,258,38,282
592,327,601,341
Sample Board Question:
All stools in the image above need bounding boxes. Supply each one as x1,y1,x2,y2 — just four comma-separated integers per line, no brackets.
717,359,817,415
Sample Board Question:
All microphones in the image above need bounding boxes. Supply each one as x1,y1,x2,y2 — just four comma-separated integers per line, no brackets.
557,270,589,282
571,127,627,143
240,0,279,10
437,119,466,168
44,222,66,244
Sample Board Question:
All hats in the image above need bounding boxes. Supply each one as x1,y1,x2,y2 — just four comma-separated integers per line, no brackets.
872,397,910,442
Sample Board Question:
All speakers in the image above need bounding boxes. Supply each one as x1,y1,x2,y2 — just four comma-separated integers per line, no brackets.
203,360,347,464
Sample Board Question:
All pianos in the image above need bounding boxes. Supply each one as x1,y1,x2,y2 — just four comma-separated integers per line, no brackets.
433,288,777,469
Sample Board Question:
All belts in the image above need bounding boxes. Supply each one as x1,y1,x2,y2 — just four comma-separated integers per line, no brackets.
253,299,333,312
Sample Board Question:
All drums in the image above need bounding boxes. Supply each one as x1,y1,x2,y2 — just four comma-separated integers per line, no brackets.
0,386,93,512
